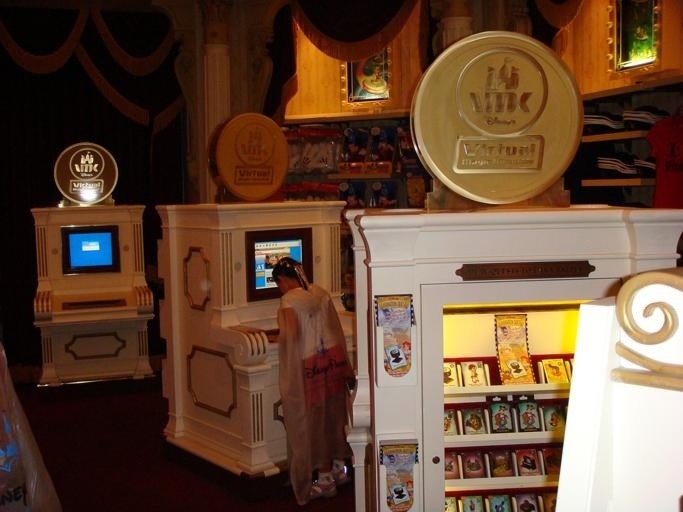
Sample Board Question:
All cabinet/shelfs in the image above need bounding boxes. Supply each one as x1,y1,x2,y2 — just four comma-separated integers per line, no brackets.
576,125,653,189
441,351,573,512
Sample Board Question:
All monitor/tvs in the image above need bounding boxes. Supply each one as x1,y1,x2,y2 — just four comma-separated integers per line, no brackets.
245,229,313,299
62,225,121,274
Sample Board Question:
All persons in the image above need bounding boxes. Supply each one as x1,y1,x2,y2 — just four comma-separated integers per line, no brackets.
266,257,355,505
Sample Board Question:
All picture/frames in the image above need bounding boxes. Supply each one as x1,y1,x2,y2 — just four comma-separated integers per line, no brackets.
606,1,663,79
336,32,400,113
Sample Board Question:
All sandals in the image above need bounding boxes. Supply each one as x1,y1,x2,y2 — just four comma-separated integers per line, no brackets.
310,468,347,497
